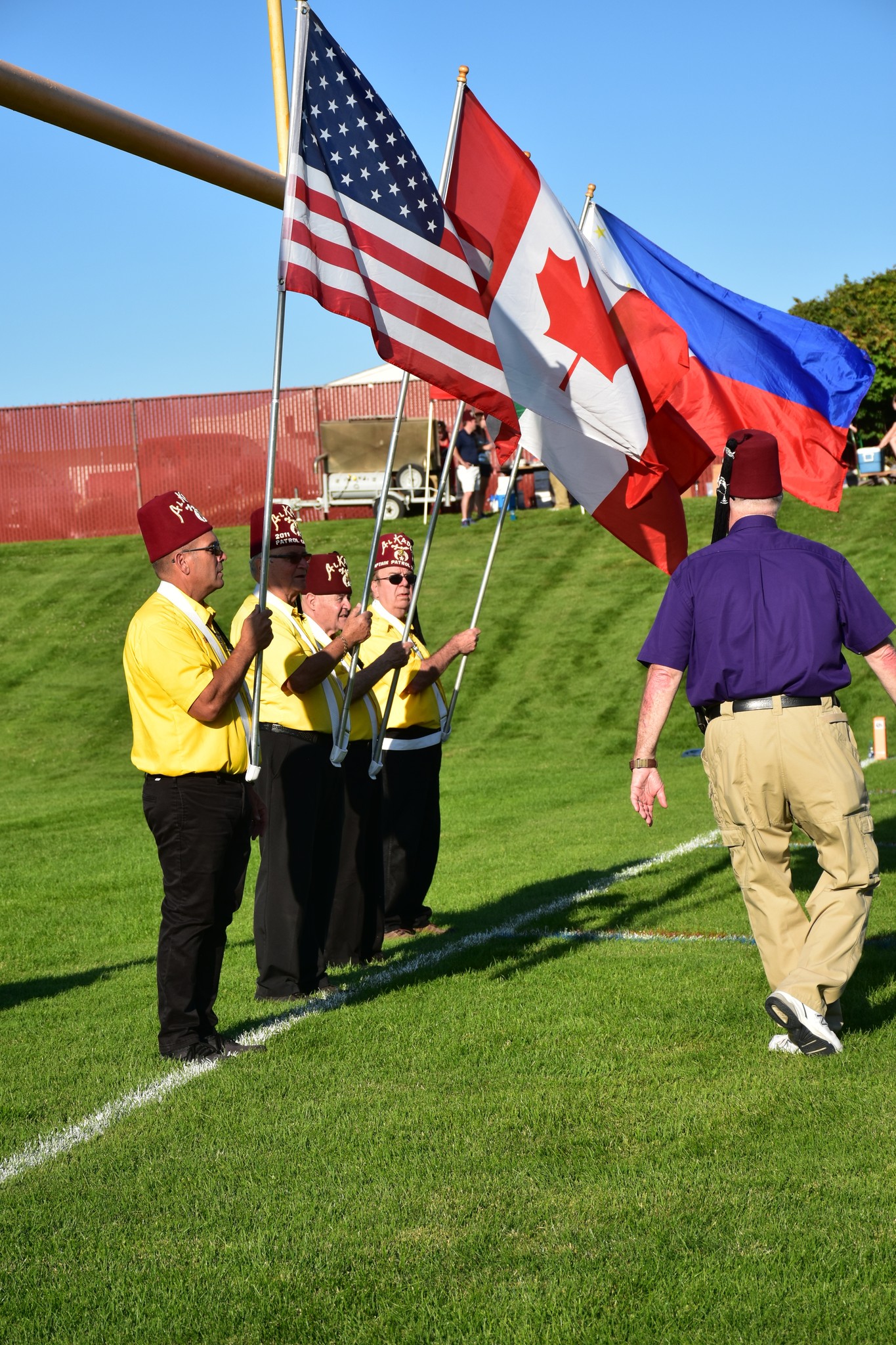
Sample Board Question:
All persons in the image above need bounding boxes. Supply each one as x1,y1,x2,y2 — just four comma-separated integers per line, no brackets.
355,530,480,939
841,424,859,487
874,394,896,471
228,504,373,1003
436,421,451,452
453,410,501,527
122,490,274,1064
629,432,896,1054
296,552,415,969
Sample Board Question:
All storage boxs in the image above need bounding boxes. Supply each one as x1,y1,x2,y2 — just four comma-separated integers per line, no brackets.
856,446,881,473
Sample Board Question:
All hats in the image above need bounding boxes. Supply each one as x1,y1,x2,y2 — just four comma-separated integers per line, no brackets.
300,551,352,596
136,489,215,564
710,429,783,545
250,504,305,622
463,410,477,422
373,532,428,645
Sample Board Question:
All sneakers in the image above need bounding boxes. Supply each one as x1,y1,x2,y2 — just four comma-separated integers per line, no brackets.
160,1041,237,1068
765,991,844,1060
203,1031,268,1061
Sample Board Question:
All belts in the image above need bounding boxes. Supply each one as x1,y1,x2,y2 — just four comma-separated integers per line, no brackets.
708,693,839,719
387,725,441,734
149,770,255,786
259,721,335,745
349,739,379,748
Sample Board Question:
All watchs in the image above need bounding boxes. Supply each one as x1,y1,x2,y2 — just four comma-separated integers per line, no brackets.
628,758,658,772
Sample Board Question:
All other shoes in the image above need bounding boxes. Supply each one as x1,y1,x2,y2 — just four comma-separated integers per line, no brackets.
254,992,328,1006
383,927,416,944
414,924,449,936
320,984,356,997
462,518,477,527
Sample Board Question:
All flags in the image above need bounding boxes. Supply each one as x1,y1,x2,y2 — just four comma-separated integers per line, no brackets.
285,0,521,469
513,398,718,577
444,84,689,509
580,200,877,514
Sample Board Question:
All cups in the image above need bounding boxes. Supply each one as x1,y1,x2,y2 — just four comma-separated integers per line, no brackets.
510,510,515,521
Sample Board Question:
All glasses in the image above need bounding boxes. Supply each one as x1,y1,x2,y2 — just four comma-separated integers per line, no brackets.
270,554,312,565
438,428,443,434
171,541,221,563
375,573,417,585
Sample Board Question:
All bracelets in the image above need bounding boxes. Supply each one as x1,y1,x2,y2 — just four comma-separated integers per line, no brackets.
336,633,349,661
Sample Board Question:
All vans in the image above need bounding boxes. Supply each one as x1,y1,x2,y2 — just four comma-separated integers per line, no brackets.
130,431,308,531
